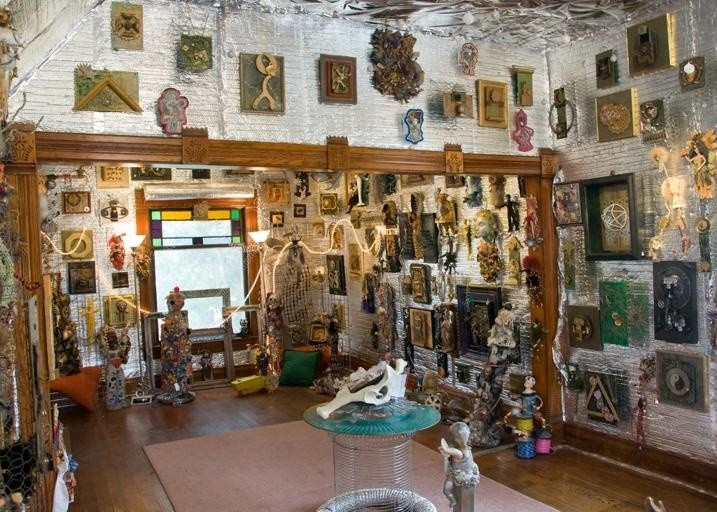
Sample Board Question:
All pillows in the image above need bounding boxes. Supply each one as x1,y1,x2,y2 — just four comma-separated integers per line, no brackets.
279,349,322,387
49,366,102,412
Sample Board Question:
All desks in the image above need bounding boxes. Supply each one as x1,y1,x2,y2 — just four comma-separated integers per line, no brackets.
302,398,442,511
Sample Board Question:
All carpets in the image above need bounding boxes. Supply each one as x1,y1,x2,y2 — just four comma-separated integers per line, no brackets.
142,419,563,512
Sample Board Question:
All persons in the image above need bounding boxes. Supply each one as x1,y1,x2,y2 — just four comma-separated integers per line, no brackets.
472,209,500,240
437,421,481,508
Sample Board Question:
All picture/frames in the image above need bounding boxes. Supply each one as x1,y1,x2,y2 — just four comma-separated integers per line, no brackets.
407,307,434,351
655,349,709,414
284,324,328,347
348,242,364,282
